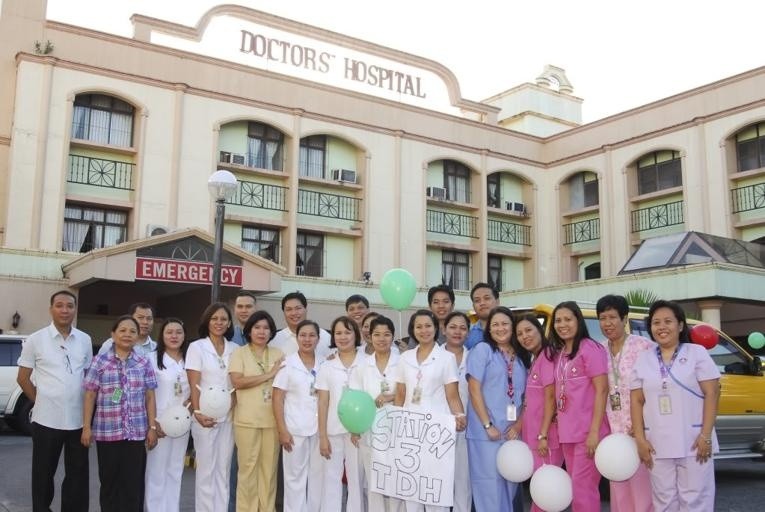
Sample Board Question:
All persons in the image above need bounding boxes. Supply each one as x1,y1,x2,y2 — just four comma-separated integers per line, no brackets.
629,300,720,510
16,292,92,512
76,283,656,510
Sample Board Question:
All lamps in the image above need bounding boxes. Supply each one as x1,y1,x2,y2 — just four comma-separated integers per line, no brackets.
11,308,22,329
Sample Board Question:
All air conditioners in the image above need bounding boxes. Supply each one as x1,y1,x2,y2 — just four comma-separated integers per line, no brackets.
329,168,357,184
505,199,526,214
222,150,246,169
424,185,448,199
143,221,168,239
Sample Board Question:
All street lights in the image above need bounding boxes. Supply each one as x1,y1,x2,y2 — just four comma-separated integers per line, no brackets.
206,169,238,304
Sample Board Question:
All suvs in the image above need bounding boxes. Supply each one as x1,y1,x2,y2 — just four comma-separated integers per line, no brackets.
466,301,765,462
0,334,35,434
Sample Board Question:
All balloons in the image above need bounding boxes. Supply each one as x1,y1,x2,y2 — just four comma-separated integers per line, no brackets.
748,332,765,350
379,267,416,312
